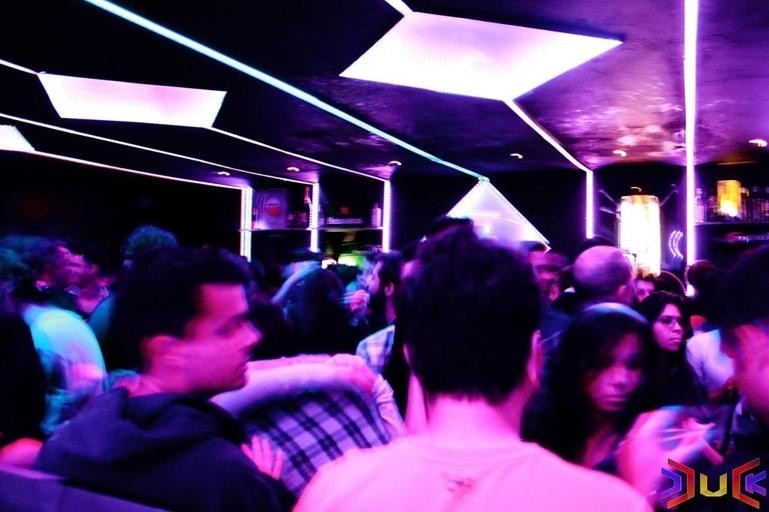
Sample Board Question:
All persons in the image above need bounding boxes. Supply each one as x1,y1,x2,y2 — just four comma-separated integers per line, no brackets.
0,212,769,512
